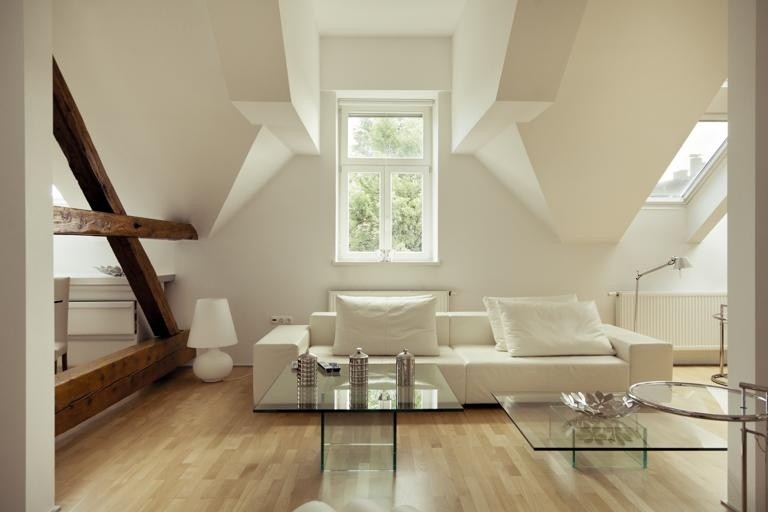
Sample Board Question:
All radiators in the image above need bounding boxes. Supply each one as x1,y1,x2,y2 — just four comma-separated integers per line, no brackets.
607,290,728,352
328,290,456,312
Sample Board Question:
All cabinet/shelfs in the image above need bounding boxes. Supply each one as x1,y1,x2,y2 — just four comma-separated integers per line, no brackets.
68,274,176,367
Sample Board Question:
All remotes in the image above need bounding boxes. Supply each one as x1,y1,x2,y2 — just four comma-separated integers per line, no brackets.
318,361,333,371
330,362,341,371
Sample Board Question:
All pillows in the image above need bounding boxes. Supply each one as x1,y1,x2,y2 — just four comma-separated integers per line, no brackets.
483,293,617,357
331,293,440,356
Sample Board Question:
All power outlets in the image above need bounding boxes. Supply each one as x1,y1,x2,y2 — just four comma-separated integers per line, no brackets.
278,316,292,325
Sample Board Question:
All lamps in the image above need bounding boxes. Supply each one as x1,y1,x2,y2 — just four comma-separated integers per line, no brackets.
186,297,239,383
633,256,694,333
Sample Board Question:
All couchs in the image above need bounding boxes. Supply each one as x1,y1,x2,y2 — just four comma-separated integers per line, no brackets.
252,312,675,411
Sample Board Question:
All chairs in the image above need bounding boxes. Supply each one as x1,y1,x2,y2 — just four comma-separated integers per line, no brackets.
54,277,71,374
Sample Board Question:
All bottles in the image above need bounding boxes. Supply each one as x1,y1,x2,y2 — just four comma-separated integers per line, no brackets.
395,348,417,386
348,347,370,386
296,347,318,388
